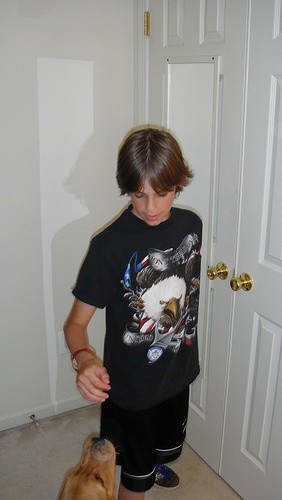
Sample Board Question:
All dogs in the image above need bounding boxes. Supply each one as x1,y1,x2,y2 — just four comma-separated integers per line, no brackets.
57,433,120,500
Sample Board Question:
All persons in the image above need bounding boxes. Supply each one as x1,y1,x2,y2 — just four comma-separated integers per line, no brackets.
64,128,203,500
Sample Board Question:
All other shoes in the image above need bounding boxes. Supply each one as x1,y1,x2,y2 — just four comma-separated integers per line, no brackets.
156,462,180,489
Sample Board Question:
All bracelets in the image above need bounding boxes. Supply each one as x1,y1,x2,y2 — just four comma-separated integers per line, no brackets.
71,348,97,373
